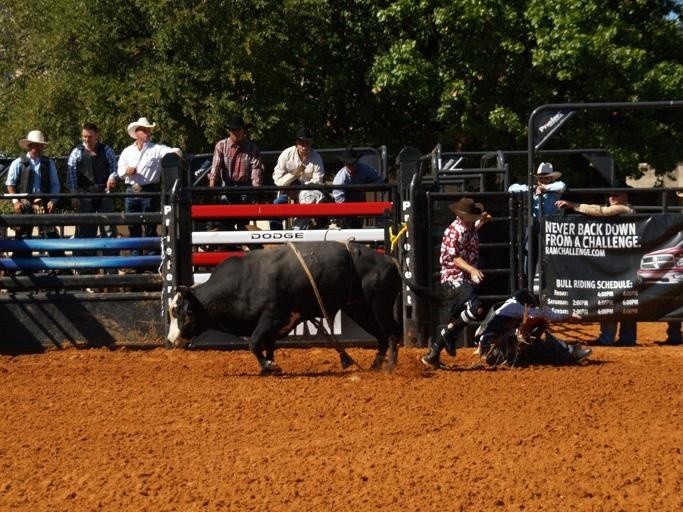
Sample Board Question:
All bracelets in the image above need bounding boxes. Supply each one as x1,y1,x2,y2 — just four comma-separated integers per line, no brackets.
11,198,18,204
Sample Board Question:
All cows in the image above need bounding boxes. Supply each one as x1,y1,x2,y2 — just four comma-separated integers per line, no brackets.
166,241,463,376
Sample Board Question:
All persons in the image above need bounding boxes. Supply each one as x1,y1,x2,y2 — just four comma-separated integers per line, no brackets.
270,132,325,230
117,117,183,274
5,130,65,274
554,180,637,347
421,198,492,369
332,150,385,229
209,117,265,231
66,123,121,276
508,162,567,280
479,288,591,368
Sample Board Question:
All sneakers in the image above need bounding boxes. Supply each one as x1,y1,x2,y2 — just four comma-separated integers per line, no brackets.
441,328,456,356
421,355,441,369
568,342,592,361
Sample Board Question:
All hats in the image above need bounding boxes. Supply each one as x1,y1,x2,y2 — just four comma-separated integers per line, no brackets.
533,162,563,179
448,197,484,218
512,289,538,308
223,116,253,129
127,117,157,139
295,128,314,144
18,130,52,150
336,150,362,165
